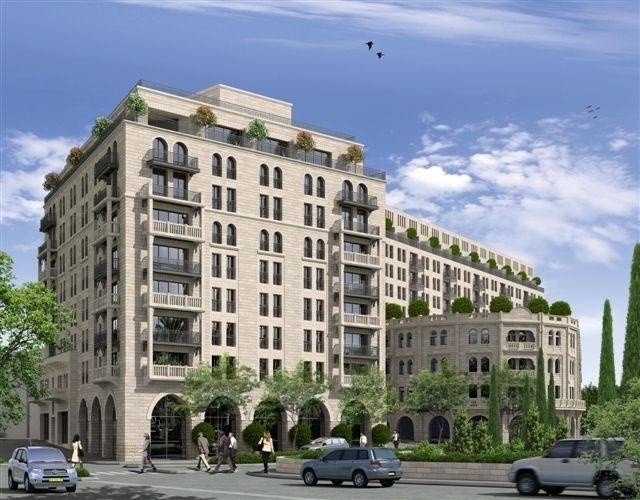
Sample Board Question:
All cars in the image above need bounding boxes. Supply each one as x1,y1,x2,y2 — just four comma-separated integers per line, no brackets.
299,436,349,455
6,446,78,493
151,422,225,452
507,435,640,500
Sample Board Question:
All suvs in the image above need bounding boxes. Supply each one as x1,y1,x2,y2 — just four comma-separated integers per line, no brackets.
299,447,403,488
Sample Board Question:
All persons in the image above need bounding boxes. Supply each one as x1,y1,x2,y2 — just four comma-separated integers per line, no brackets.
70,434,84,468
210,428,235,474
258,431,275,473
196,432,211,472
227,432,238,472
359,432,368,449
139,432,157,473
392,430,401,449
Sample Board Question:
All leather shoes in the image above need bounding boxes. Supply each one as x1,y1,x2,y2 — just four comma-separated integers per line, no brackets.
194,468,211,472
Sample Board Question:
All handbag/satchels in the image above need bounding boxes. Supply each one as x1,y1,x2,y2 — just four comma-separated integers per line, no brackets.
257,443,263,449
78,447,84,457
